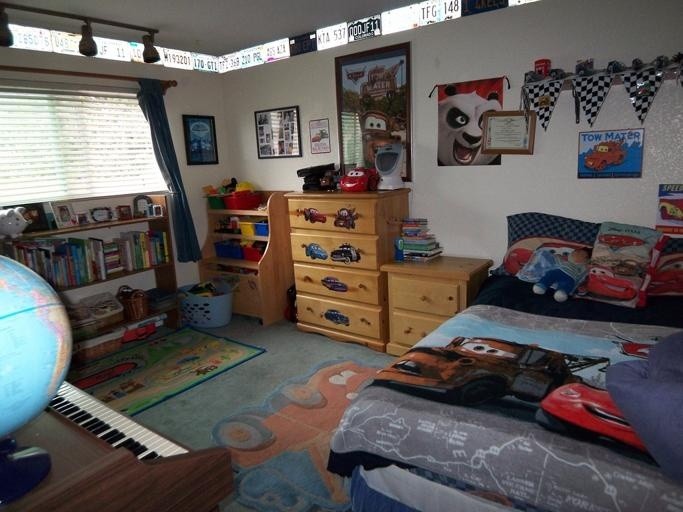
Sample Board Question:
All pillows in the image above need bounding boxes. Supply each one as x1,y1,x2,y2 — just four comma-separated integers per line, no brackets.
493,207,683,310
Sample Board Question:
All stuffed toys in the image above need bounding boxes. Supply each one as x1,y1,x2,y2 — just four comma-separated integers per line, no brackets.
532,246,591,304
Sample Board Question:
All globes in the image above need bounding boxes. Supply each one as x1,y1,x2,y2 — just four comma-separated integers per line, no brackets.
0,254,73,503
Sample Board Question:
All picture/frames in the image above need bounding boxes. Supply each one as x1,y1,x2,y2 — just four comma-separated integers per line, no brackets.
254,105,303,159
182,114,218,165
334,40,414,184
48,199,78,228
3,202,50,233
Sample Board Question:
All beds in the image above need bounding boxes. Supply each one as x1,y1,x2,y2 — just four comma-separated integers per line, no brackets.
329,226,683,511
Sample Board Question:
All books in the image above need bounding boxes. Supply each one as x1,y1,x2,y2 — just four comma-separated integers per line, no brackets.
116,203,133,223
2,228,178,341
400,216,443,264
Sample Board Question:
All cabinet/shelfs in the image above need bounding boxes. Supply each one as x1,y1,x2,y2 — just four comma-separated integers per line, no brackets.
0,193,182,361
195,187,294,326
283,188,409,354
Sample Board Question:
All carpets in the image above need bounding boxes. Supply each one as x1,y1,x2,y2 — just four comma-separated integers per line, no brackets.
65,326,267,416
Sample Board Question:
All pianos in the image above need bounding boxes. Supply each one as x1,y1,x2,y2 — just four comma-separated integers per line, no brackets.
0,380,237,511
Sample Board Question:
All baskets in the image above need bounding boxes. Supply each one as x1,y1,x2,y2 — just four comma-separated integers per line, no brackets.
116,285,150,322
68,302,97,341
78,291,124,331
176,277,234,329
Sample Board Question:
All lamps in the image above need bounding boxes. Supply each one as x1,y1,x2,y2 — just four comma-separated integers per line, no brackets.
142,30,160,65
80,19,97,57
1,4,14,47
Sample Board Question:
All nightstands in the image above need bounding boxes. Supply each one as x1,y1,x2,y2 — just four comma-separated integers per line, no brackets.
378,256,494,358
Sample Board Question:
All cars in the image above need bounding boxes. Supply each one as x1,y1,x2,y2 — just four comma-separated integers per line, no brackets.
305,243,327,260
331,243,360,265
302,207,326,224
321,277,347,292
583,140,625,171
535,379,647,458
588,265,634,299
372,336,610,408
325,308,350,326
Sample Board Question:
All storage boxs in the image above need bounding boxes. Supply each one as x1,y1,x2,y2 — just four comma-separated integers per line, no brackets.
208,190,269,262
